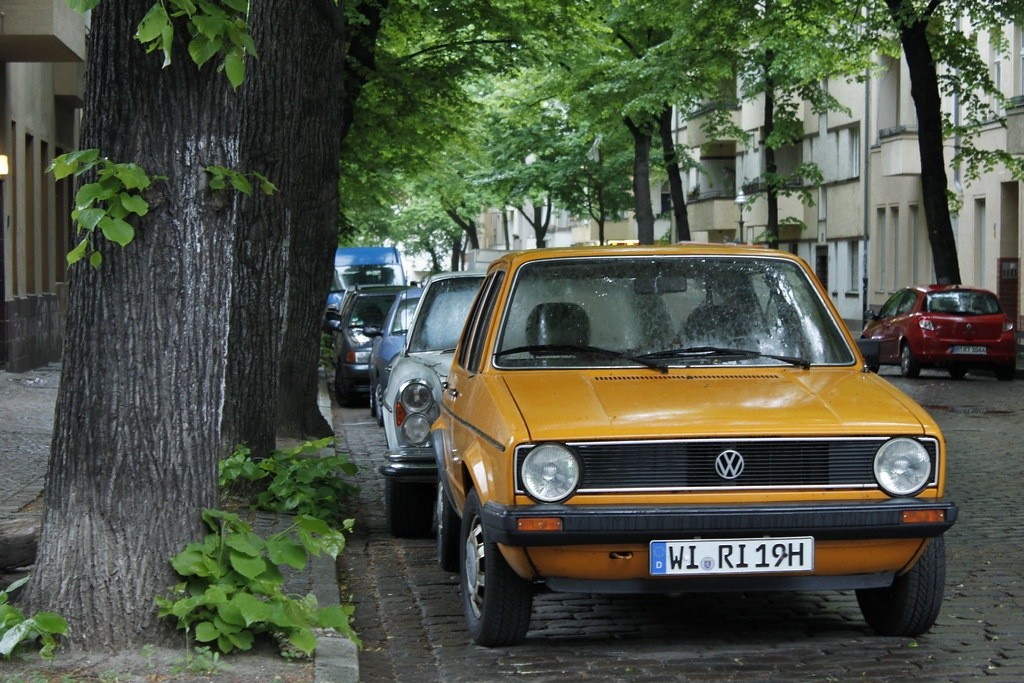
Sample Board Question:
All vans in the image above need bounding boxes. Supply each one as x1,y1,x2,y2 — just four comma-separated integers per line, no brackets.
322,245,410,334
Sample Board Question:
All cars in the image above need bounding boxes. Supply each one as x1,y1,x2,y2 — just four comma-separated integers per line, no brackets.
860,284,1017,380
323,285,406,408
361,286,424,427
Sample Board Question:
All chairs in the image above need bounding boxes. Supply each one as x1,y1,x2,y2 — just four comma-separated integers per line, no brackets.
525,299,774,361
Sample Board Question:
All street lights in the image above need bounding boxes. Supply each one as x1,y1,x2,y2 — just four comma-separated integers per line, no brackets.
734,190,750,245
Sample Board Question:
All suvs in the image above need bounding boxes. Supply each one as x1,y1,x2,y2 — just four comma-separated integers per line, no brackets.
382,272,490,539
430,241,960,648
